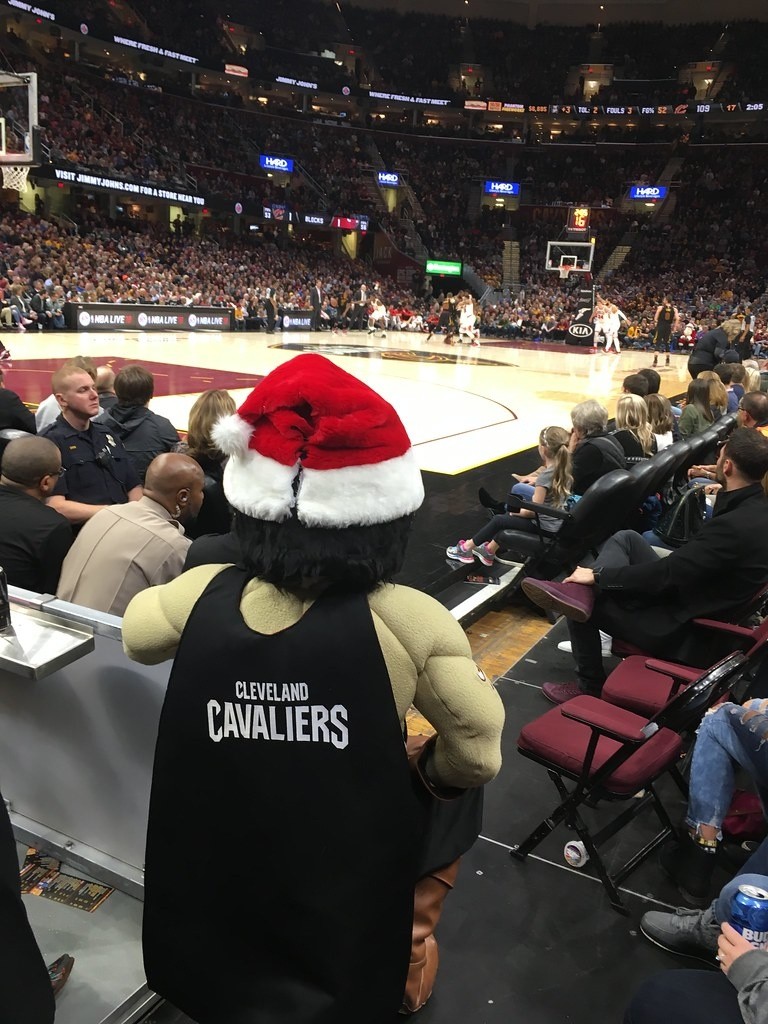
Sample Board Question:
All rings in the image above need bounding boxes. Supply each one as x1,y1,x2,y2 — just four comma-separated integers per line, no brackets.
717,955,725,963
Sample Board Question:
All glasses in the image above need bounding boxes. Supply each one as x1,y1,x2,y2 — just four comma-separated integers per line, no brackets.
737,406,745,412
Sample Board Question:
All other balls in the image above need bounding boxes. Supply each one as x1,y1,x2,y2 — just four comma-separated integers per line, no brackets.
331,299,337,306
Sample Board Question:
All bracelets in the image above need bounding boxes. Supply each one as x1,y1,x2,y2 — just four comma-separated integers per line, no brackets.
706,471,712,479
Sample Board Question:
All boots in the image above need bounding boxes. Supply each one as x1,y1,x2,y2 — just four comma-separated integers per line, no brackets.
511,465,547,486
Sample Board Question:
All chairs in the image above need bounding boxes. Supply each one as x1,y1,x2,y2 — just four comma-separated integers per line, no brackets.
486,411,738,626
509,585,768,915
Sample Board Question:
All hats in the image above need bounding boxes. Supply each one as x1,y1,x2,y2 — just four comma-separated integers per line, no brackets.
210,352,427,527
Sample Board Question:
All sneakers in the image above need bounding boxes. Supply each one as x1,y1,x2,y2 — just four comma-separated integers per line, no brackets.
558,631,613,658
522,576,593,619
446,540,495,568
664,841,716,906
640,900,729,969
480,488,508,519
542,681,589,705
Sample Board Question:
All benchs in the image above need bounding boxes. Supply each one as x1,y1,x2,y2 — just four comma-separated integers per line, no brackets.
0,584,174,900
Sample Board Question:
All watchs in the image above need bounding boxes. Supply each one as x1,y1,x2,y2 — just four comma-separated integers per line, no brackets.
592,565,605,584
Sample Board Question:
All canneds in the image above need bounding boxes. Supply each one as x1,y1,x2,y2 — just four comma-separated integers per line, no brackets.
727,884,768,950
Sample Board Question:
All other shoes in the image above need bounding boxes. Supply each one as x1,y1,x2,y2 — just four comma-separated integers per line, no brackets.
457,339,462,344
311,328,315,331
470,343,480,348
316,328,322,331
22,317,33,325
10,326,18,330
380,332,387,339
342,328,348,337
652,355,658,367
267,330,274,334
332,328,339,335
0,326,7,330
665,354,669,366
18,323,26,330
55,323,67,328
368,328,375,335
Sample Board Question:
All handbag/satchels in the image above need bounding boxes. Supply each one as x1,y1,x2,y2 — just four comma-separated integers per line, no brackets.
654,487,708,544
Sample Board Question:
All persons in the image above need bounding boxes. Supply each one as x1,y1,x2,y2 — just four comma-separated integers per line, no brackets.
446,321,767,565
1,23,768,356
122,353,505,1024
640,699,768,1024
0,357,236,615
649,296,680,368
519,428,768,704
